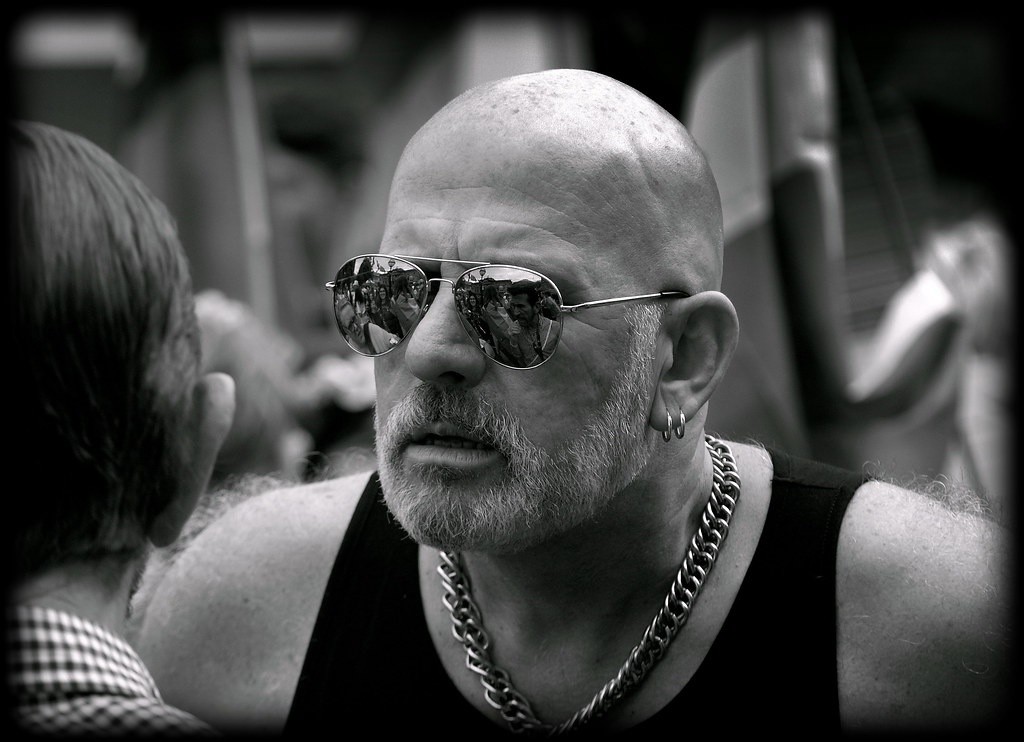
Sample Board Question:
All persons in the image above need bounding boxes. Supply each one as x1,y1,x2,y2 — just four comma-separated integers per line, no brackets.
0,121,236,742
133,65,1019,742
190,286,377,524
336,272,422,353
459,278,562,367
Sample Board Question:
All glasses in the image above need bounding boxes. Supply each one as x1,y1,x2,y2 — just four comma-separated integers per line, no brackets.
325,254,690,371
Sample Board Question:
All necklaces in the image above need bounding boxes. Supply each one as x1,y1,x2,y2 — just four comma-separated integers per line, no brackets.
434,432,742,740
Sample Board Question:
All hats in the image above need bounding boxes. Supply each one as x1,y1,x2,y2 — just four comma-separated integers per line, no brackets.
353,280,359,286
